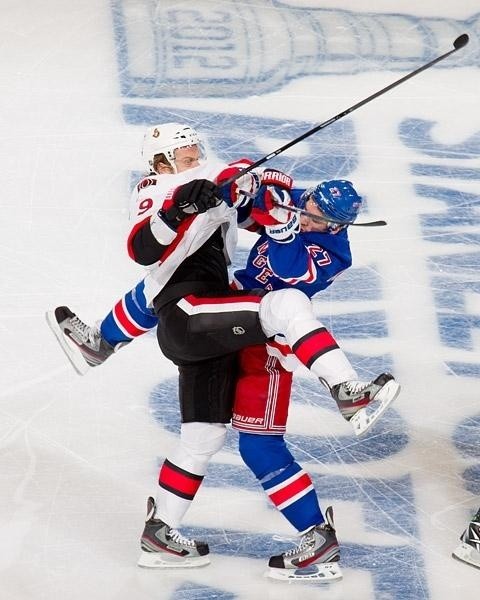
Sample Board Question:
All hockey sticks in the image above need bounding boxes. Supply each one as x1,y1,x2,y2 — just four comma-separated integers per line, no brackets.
236,188,386,226
218,35,468,189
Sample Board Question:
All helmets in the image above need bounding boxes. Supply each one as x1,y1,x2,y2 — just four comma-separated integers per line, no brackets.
300,179,362,235
141,121,200,175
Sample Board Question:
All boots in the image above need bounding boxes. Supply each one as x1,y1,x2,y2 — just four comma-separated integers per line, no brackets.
268,505,341,568
139,496,210,559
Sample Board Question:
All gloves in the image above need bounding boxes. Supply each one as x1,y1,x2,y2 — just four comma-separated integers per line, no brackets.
249,183,299,240
162,179,225,228
215,157,263,209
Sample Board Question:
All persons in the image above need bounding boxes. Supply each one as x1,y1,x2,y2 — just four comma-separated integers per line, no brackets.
126,122,403,571
44,177,360,583
452,504,479,572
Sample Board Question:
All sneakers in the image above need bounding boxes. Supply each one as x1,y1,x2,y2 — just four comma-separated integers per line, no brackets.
317,369,396,422
54,305,134,368
460,508,480,552
